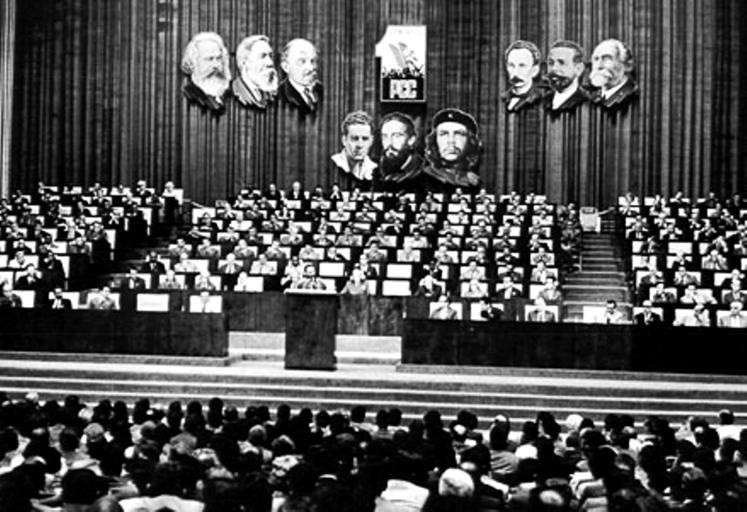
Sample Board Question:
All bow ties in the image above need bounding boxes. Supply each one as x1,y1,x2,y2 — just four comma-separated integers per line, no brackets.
510,90,528,100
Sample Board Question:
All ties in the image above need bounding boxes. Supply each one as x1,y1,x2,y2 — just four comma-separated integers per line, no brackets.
304,88,313,104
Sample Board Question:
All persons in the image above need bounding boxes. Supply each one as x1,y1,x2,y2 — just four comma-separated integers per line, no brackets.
418,107,483,186
545,41,585,116
498,40,550,112
275,36,324,111
580,38,641,110
374,112,422,184
328,109,377,182
227,34,279,109
181,31,231,112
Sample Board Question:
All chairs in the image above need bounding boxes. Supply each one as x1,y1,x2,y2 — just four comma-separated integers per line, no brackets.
582,303,635,328
557,202,585,274
616,194,747,330
0,182,564,330
578,205,602,236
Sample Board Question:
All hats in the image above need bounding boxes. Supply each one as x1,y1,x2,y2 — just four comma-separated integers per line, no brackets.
432,108,478,133
